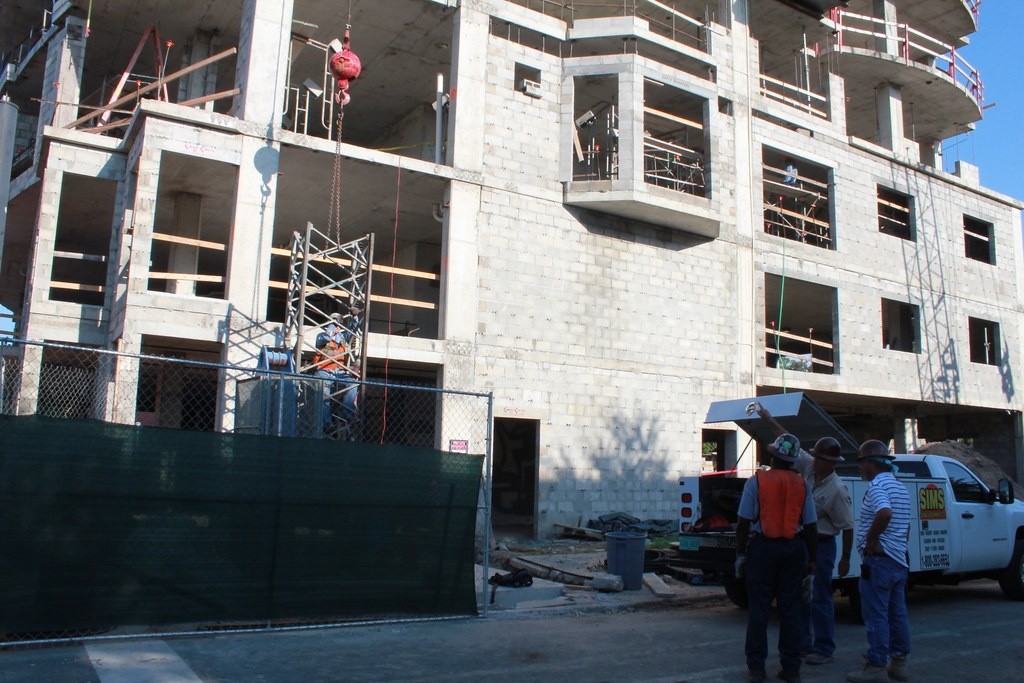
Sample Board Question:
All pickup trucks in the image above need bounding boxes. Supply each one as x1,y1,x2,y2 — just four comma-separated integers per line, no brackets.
675,385,1022,617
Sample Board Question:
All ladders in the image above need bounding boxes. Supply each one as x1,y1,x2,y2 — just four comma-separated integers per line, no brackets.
92,24,169,138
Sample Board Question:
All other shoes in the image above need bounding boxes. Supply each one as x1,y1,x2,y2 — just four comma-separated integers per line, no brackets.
888,655,909,681
777,670,801,683
805,652,835,665
747,668,767,683
845,662,889,683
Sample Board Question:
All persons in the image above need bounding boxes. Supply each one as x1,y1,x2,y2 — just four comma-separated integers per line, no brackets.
734,431,819,682
757,400,853,665
312,317,361,429
783,153,799,187
846,439,911,682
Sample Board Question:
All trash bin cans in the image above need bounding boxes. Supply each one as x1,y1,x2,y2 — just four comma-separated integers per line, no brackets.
606,531,648,591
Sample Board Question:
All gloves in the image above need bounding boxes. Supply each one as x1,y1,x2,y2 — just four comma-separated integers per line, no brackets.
734,550,745,579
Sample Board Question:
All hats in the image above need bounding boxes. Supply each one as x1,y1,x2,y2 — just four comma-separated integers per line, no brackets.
766,433,803,462
808,436,846,462
330,312,344,325
856,439,897,462
